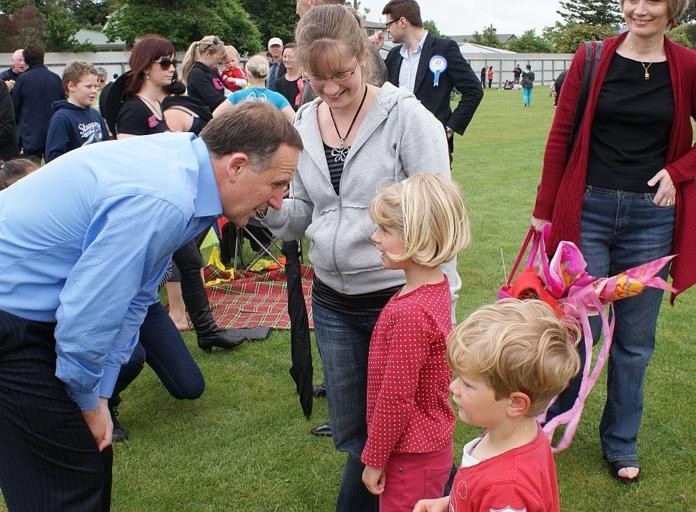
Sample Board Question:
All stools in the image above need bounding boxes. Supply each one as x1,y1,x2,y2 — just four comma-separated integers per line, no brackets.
232,216,303,277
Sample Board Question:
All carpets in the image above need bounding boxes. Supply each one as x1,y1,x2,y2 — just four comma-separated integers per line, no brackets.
164,259,318,329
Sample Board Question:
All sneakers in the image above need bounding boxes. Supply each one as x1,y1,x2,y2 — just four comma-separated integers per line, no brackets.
108,406,128,441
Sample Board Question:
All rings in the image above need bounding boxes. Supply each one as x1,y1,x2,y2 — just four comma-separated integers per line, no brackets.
665,199,671,205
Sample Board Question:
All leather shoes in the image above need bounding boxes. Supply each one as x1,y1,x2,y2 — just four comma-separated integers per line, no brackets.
311,424,334,436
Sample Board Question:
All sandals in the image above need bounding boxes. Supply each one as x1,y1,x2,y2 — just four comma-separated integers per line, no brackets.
607,458,641,484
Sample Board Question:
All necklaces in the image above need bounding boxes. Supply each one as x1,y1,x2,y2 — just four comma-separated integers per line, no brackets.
330,84,367,162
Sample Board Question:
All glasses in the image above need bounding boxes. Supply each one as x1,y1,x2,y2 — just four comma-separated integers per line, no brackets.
301,64,356,87
149,58,178,70
385,19,397,28
205,35,220,50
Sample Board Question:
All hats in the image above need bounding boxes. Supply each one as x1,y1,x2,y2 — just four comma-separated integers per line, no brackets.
98,69,132,137
268,37,283,49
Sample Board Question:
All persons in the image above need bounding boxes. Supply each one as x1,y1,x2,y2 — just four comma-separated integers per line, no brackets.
1,99,303,510
480,67,487,89
488,66,494,89
246,0,463,512
2,34,319,442
549,79,555,98
296,0,387,437
369,0,484,169
512,64,522,84
521,64,534,107
413,296,581,512
631,38,661,80
530,0,696,484
359,173,470,512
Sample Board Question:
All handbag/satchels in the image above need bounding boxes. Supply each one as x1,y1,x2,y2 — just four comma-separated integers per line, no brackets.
497,222,543,305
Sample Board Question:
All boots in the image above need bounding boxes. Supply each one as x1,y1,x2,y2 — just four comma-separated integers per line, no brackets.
183,289,247,352
